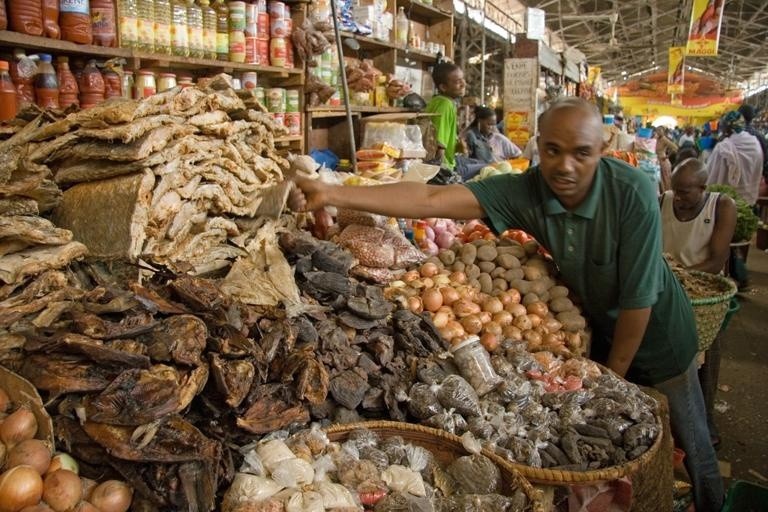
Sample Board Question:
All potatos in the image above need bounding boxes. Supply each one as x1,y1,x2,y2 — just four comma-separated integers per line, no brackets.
424,236,587,332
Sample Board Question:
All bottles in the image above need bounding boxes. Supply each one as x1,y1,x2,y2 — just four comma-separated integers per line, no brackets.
396,5,411,46
450,336,505,398
0,0,247,130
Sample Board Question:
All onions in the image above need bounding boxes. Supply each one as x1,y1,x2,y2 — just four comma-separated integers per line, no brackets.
1,387,135,512
384,261,582,351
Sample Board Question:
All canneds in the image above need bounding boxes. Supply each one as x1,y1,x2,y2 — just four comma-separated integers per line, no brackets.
228,0,302,135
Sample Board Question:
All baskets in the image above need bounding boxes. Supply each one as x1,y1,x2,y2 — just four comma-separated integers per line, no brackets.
479,354,666,486
665,270,737,351
219,420,541,512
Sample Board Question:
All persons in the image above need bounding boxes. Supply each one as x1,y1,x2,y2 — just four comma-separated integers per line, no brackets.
458,105,522,164
657,156,737,276
282,97,725,511
646,104,768,216
424,63,489,184
